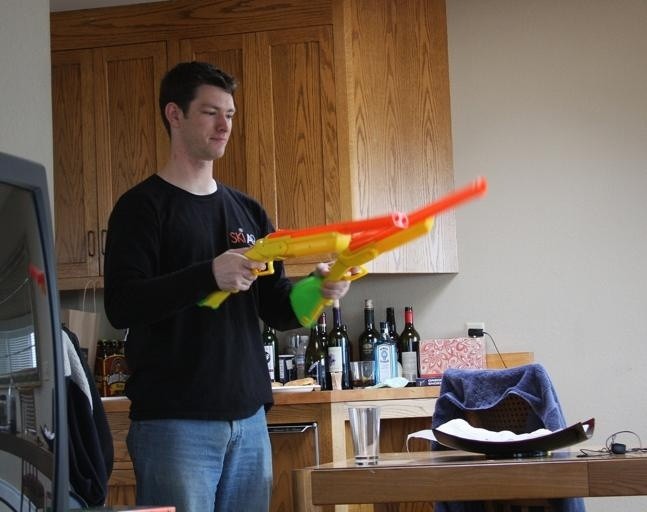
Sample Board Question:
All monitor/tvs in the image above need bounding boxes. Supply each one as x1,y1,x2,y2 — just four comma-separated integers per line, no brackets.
0,152,70,511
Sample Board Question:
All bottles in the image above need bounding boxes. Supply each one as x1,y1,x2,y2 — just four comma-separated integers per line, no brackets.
260,321,278,381
375,323,398,385
305,298,351,391
359,299,379,359
384,306,399,346
96,338,125,358
290,332,304,378
398,306,421,385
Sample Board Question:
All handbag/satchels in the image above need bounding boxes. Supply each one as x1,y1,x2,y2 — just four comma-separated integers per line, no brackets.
64,280,100,377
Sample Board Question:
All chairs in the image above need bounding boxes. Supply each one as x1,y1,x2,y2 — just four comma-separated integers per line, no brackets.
428,366,590,511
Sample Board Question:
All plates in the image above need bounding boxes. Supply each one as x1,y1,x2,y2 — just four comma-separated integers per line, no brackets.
269,384,321,393
430,418,596,457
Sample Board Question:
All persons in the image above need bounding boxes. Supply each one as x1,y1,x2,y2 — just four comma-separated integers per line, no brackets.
102,59,362,508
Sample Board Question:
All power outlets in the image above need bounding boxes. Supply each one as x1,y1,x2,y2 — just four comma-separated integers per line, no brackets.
466,323,487,340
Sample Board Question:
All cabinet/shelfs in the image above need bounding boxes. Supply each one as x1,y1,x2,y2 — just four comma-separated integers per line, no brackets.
174,0,459,278
49,1,171,285
94,353,533,512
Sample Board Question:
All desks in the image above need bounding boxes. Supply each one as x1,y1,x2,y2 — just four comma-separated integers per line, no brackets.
293,446,647,512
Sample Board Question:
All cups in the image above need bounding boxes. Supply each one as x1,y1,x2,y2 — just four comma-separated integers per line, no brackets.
347,404,381,467
286,335,309,353
277,354,297,384
349,360,376,387
